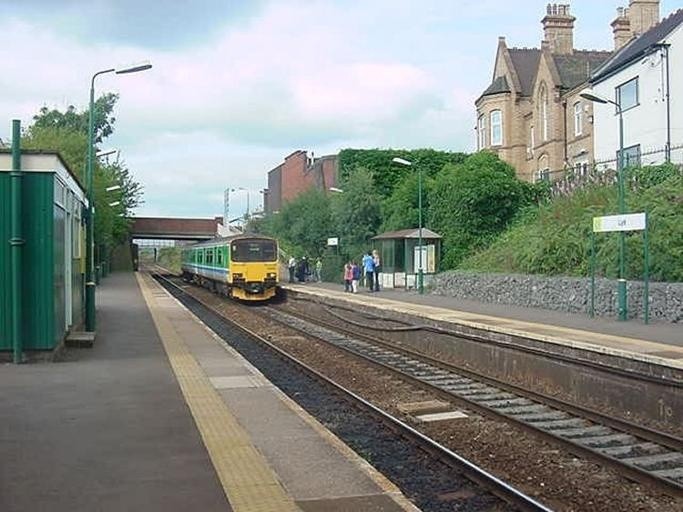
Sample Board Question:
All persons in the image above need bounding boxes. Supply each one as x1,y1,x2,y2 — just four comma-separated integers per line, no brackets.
344,260,353,293
372,249,381,292
352,260,361,294
288,255,323,284
364,252,375,290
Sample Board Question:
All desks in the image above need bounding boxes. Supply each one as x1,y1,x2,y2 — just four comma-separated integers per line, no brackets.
579,92,628,321
85,63,153,332
391,156,424,295
